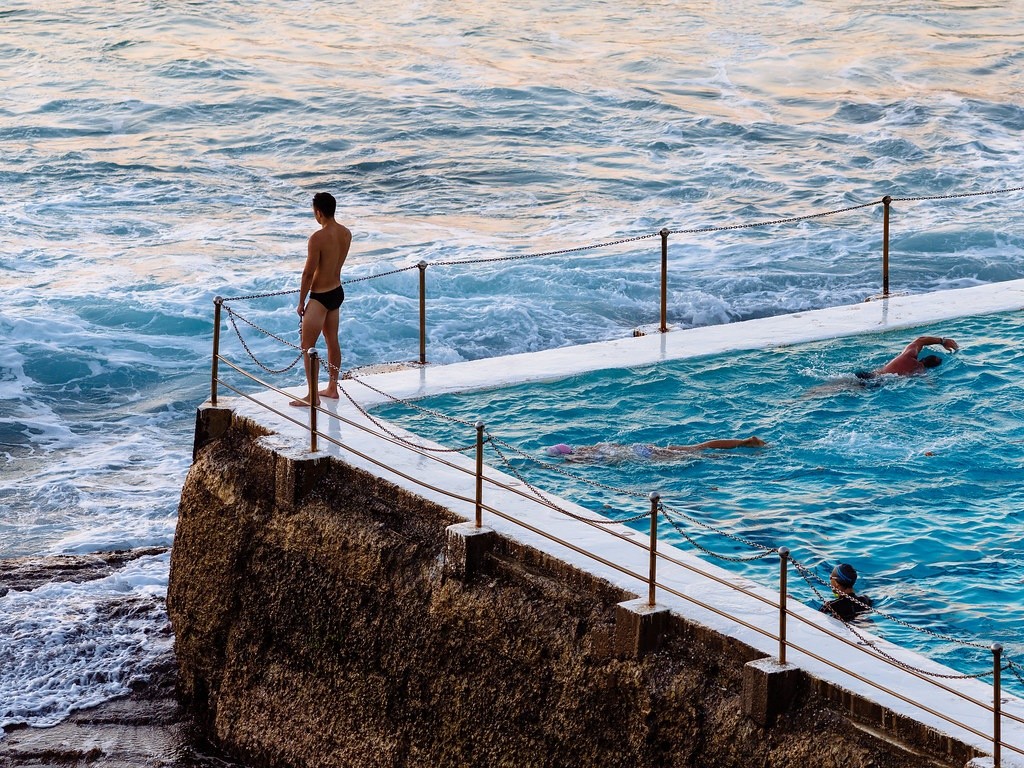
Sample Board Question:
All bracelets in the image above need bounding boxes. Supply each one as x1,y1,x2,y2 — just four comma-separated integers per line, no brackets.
941,338,945,344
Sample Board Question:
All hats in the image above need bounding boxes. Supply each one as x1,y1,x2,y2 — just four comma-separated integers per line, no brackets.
548,444,573,456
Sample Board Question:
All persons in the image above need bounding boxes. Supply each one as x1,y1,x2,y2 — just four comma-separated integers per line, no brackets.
818,564,872,623
856,336,958,387
555,436,765,454
288,193,352,406
